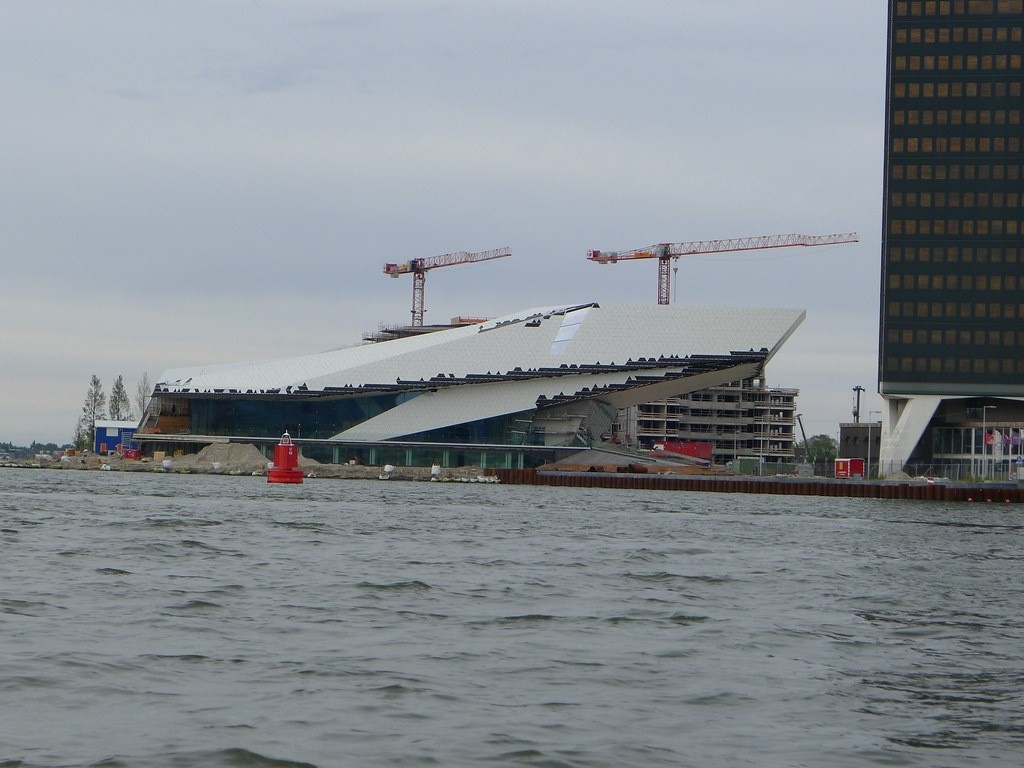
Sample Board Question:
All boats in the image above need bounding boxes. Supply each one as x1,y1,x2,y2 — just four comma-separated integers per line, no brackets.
379,475,390,480
252,471,263,476
432,475,501,484
308,473,316,478
154,466,167,472
101,464,111,470
230,469,241,474
0,463,63,469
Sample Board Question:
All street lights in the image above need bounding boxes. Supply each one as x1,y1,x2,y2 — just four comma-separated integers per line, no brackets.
982,405,997,481
868,411,882,479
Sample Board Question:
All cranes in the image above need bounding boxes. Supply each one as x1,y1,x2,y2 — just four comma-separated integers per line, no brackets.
384,246,510,328
851,386,865,423
587,233,859,306
